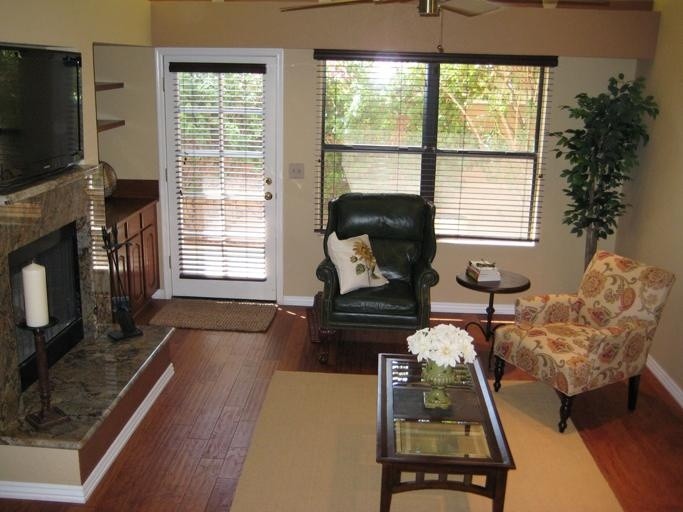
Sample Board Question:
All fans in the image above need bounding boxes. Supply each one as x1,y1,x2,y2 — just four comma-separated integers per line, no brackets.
279,0,501,54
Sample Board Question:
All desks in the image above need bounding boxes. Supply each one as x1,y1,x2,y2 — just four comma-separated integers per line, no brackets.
456,269,531,371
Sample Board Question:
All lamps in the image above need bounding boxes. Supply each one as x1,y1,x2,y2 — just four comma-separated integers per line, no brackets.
417,1,445,53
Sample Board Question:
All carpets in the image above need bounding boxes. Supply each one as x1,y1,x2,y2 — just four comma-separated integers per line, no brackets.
148,299,277,333
228,370,624,512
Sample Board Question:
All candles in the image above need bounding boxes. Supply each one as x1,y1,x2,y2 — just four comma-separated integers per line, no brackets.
22,257,50,329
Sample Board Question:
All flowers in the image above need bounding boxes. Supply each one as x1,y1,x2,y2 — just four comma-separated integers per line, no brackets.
405,322,477,365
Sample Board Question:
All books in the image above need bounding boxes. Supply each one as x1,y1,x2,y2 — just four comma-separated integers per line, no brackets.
464,258,501,282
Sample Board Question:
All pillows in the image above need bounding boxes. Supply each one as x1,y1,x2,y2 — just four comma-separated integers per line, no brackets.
326,232,389,296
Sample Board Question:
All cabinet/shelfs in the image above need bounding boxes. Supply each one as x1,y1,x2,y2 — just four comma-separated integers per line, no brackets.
104,222,131,327
126,201,161,319
93,82,125,133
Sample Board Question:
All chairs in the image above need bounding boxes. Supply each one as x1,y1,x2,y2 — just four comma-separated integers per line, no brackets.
494,249,677,432
308,192,439,365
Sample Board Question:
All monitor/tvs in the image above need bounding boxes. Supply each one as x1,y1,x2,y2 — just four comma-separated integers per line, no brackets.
0,41,84,197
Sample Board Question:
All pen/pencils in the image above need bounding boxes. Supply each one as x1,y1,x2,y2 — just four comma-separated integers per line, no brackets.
394,418,430,423
440,420,480,424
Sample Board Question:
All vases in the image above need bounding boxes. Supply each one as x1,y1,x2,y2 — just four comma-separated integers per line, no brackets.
421,359,457,411
